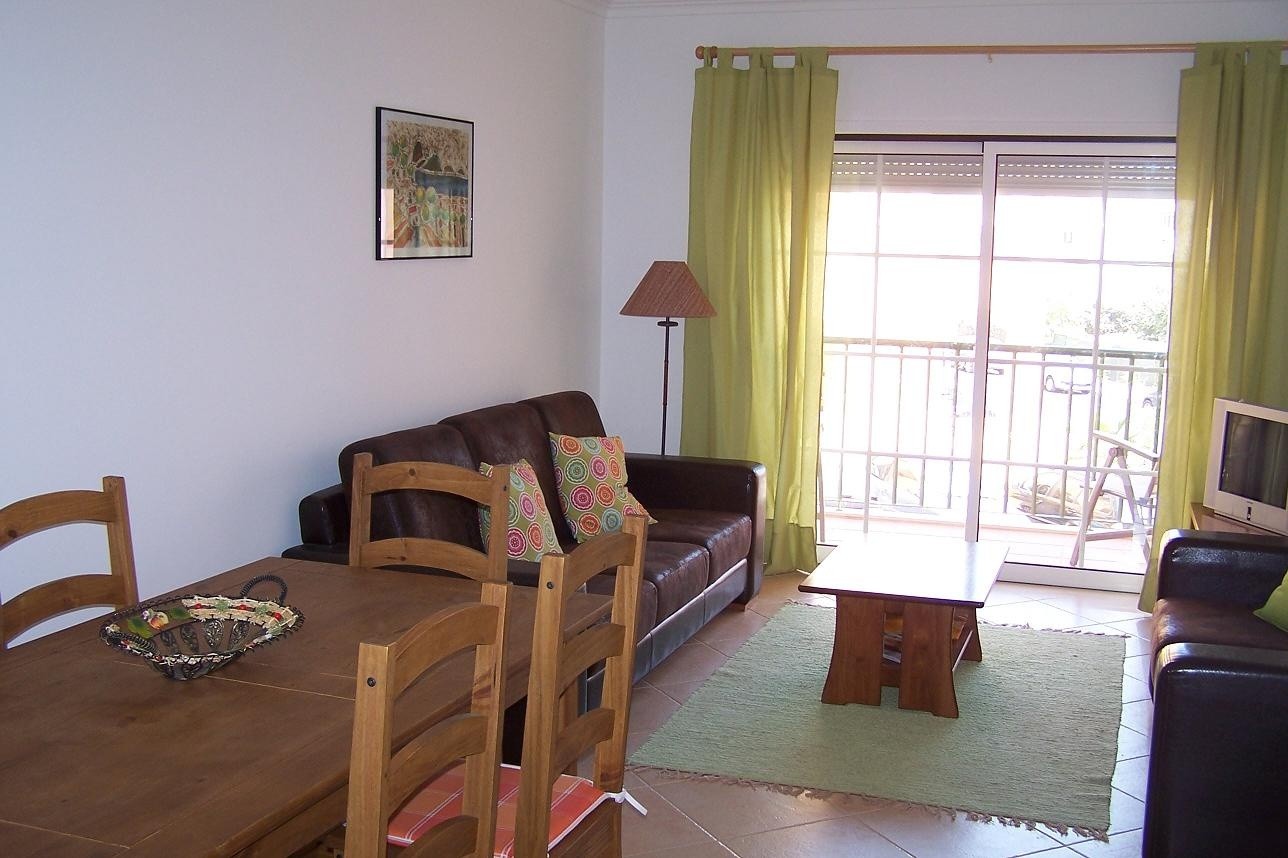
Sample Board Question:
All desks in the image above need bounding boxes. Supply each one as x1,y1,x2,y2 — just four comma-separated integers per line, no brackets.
0,556,615,858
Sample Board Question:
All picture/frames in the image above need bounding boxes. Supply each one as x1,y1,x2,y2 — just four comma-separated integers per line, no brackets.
375,107,473,261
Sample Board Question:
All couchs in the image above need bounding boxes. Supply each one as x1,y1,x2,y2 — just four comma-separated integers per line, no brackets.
282,391,766,711
1141,530,1288,858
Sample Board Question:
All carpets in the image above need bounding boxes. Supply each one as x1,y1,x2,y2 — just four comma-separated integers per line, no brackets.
625,598,1133,842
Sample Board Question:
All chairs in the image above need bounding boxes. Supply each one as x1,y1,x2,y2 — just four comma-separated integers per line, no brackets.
1070,429,1158,567
349,453,510,582
324,515,649,858
0,475,138,649
301,578,511,858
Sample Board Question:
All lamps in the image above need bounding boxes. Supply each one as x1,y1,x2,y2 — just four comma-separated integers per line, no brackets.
619,260,717,455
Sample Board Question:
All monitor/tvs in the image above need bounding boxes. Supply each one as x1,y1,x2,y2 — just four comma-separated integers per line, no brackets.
1203,397,1288,536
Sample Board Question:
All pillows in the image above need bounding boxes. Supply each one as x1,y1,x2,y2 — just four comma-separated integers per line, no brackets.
548,432,659,543
477,459,563,562
1253,571,1288,633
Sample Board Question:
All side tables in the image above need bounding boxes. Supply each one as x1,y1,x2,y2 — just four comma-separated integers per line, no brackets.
1189,502,1274,535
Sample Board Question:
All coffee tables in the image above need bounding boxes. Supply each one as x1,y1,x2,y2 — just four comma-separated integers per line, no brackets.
798,533,1011,719
